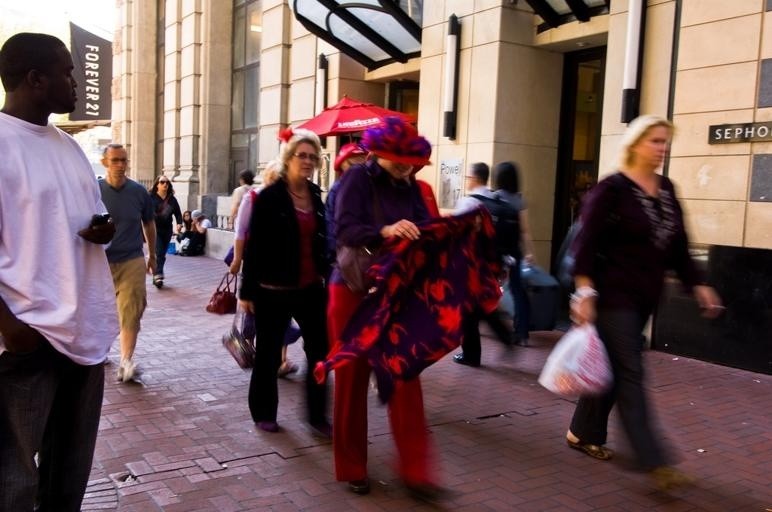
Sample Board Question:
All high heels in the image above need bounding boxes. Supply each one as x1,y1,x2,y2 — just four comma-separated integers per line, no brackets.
403,476,455,500
338,478,367,495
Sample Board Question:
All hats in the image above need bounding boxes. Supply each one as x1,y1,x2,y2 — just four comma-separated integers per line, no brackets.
334,145,364,170
192,209,203,219
362,114,432,166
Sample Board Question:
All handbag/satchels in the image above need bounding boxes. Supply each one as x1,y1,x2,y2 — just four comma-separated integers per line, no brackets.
334,164,386,292
205,268,239,315
220,301,259,370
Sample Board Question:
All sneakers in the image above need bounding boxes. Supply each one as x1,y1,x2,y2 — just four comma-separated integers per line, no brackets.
116,360,143,386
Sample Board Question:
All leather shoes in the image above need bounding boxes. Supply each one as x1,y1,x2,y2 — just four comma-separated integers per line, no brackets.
251,416,280,435
296,410,335,440
451,353,483,370
152,276,166,291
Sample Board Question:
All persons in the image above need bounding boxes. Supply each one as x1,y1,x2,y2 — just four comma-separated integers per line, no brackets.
228,155,298,374
232,131,350,436
1,31,122,509
144,174,182,290
89,146,147,384
177,211,192,243
558,113,721,486
227,169,251,225
325,116,466,507
335,141,366,176
435,161,519,366
182,211,211,256
484,162,531,346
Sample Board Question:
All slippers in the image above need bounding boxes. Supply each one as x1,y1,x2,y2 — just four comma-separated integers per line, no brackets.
645,464,700,493
566,428,615,464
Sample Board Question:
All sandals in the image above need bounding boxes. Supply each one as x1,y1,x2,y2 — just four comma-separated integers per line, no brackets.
280,353,297,377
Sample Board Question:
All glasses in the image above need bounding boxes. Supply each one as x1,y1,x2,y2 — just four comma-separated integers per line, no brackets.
291,150,317,161
158,180,170,185
106,157,129,165
462,174,476,179
